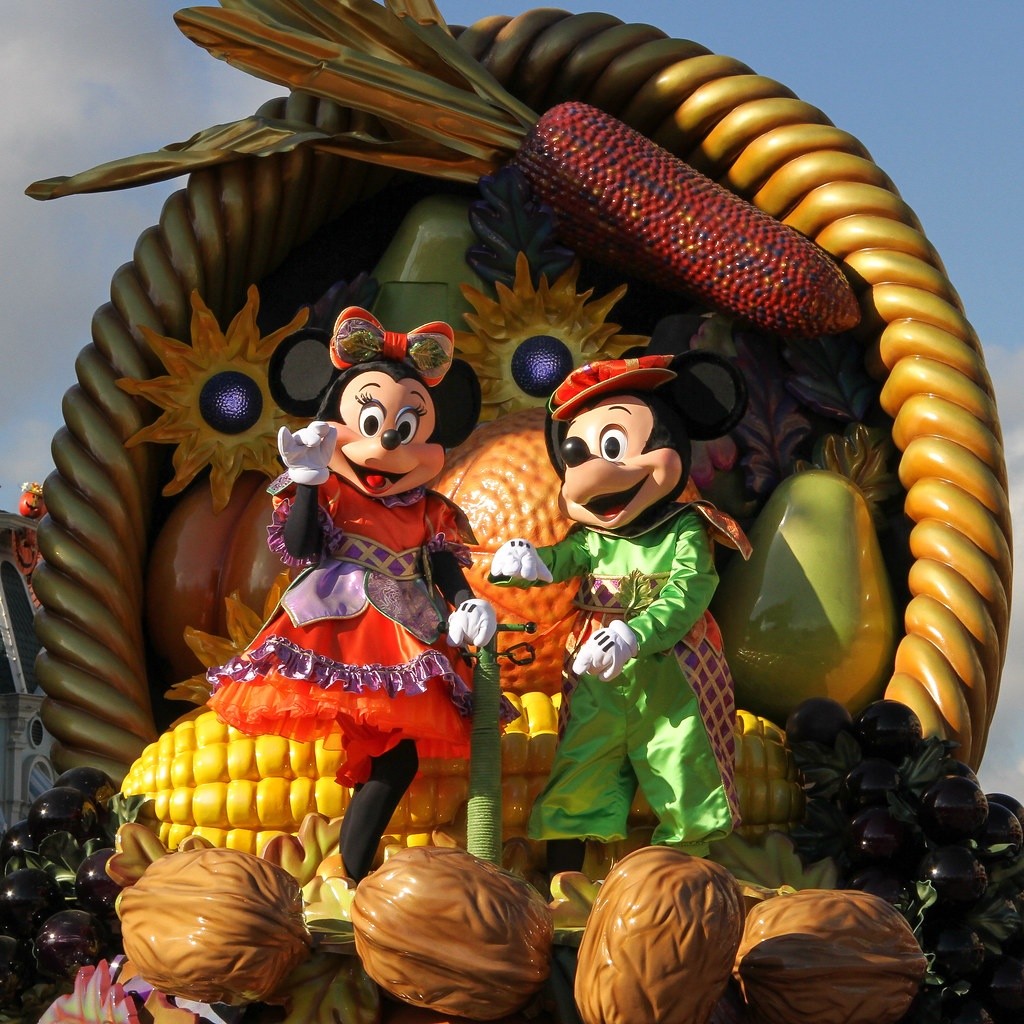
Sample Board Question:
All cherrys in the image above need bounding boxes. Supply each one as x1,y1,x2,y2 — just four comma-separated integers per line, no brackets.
1,765,117,1024
784,698,1023,1022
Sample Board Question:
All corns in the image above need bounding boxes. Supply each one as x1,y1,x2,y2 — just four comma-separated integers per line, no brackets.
23,0,863,340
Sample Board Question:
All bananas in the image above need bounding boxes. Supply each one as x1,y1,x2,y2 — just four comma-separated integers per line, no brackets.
146,471,298,693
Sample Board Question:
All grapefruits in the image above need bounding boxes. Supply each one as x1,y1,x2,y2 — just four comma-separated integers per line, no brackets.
713,469,896,719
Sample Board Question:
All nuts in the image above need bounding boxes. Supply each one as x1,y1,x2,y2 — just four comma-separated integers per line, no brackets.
117,848,931,1022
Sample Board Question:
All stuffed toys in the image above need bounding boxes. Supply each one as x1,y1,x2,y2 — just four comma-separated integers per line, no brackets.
487,353,756,893
207,309,498,884
